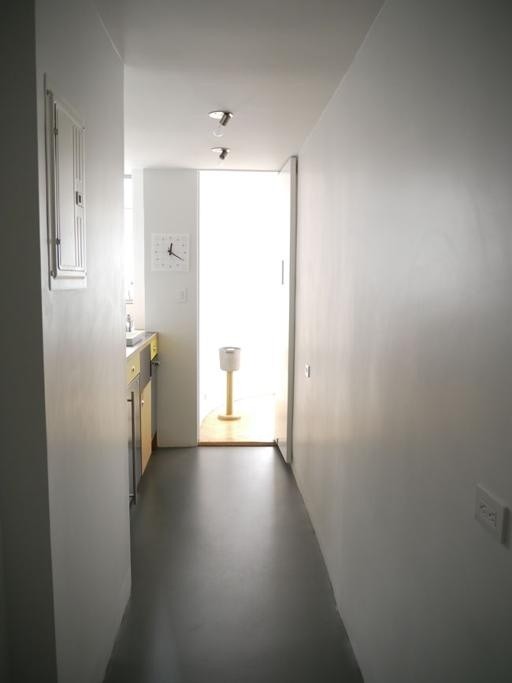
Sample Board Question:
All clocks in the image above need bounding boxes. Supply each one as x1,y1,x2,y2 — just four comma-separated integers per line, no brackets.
150,233,190,271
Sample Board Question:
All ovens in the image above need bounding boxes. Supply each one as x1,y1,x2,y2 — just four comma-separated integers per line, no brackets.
127,373,143,509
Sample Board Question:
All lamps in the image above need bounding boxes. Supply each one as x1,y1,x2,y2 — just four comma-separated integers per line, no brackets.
211,146,231,159
209,110,234,136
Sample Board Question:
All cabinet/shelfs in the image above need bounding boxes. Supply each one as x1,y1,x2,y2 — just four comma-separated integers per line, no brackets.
126,331,159,502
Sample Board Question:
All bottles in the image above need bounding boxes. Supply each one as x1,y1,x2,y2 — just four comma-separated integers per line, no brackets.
125,312,136,332
